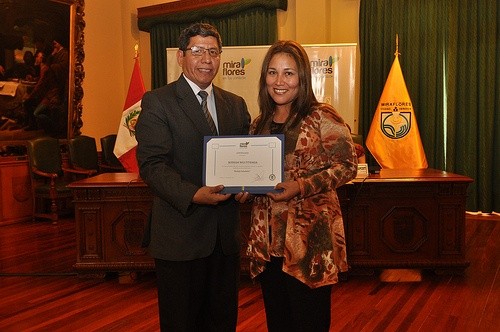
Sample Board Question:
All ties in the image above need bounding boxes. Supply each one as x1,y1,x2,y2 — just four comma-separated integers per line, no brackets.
198,91,218,136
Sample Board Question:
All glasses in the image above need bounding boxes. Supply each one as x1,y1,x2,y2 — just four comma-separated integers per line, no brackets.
185,45,222,57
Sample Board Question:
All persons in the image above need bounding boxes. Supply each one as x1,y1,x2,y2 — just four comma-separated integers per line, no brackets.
236,40,358,332
345,123,365,164
135,23,251,332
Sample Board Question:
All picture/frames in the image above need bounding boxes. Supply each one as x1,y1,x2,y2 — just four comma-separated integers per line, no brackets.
0,0,86,158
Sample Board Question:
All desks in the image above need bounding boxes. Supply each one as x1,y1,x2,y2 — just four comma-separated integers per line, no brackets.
65,166,475,280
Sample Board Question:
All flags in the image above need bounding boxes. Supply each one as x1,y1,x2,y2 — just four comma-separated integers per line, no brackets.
366,52,428,169
113,52,146,172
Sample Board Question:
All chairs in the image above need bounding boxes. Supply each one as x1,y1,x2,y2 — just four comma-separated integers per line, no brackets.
70,134,121,177
26,137,92,222
101,135,125,172
